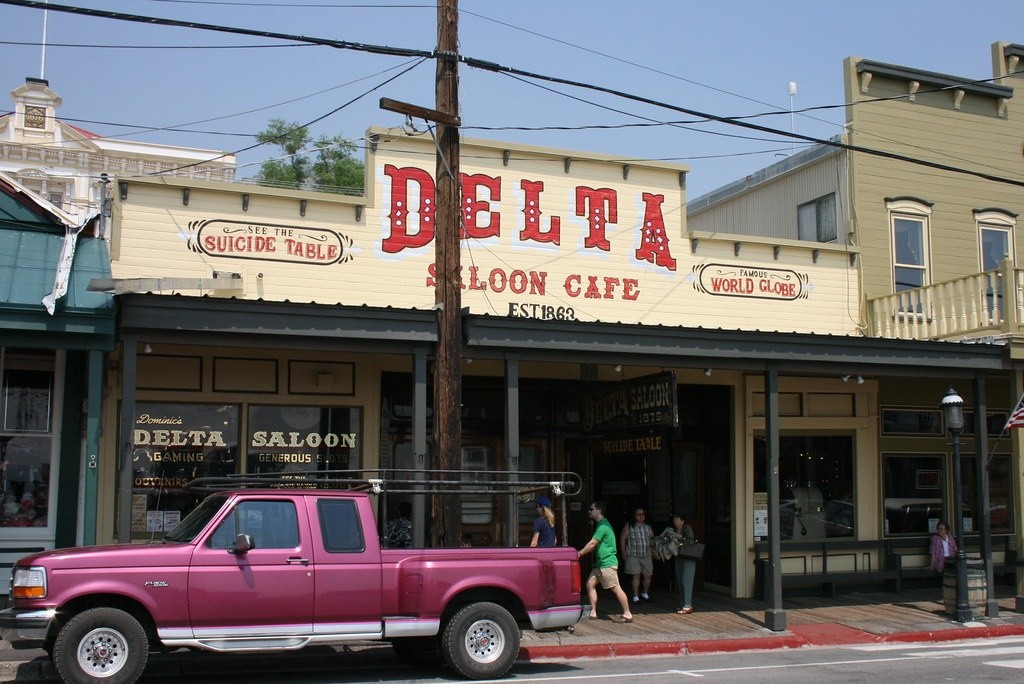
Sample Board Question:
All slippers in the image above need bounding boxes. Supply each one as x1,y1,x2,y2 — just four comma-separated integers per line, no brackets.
612,615,634,623
589,613,597,620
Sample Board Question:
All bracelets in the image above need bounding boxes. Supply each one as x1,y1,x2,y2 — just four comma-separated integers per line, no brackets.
578,551,582,558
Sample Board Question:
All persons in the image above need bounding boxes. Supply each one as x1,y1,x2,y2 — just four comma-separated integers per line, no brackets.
459,533,475,549
924,522,957,572
531,495,558,550
620,505,655,603
669,512,694,614
577,500,633,623
387,502,413,549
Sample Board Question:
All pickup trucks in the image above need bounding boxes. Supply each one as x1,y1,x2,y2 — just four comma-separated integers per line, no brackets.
0,472,583,684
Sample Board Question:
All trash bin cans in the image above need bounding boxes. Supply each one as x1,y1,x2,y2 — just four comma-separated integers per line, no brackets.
944,555,988,618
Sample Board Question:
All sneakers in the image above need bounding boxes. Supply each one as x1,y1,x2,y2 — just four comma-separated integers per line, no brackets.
676,607,693,614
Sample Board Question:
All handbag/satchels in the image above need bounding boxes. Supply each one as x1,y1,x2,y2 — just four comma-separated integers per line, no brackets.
678,538,705,560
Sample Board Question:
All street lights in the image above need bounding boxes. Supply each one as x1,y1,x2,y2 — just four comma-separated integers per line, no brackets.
942,388,975,624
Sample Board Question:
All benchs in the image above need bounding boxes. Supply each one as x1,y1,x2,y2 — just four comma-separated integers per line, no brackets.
886,535,1019,595
753,540,897,601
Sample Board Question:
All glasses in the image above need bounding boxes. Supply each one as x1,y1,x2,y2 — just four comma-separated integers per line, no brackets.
638,513,644,516
536,505,543,508
589,508,595,511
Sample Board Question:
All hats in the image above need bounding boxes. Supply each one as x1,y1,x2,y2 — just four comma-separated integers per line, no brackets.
536,496,551,506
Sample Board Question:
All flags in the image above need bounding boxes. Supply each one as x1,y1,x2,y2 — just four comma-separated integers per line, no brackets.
1003,394,1024,430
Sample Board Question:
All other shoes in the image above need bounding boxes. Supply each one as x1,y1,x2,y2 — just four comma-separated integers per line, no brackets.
632,594,641,604
640,593,653,604
